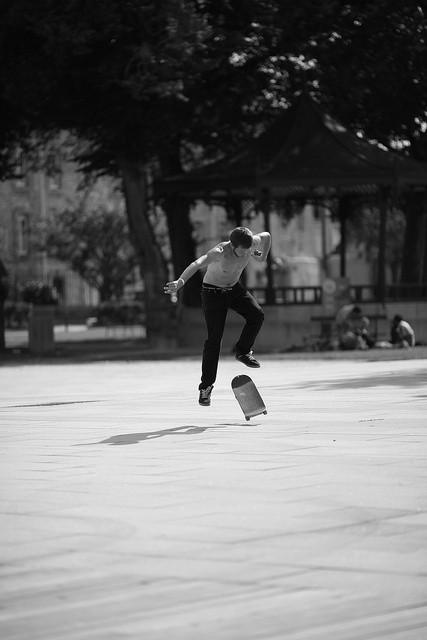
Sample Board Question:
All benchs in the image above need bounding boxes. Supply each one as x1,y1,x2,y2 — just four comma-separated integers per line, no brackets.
310,316,389,348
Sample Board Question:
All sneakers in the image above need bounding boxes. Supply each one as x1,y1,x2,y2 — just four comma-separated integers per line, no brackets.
235,352,260,368
198,384,214,406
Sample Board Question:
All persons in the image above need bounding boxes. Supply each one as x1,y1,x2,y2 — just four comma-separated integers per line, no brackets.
335,304,361,349
391,313,415,348
164,226,271,406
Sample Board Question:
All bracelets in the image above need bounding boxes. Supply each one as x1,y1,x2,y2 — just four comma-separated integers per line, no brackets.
179,278,185,285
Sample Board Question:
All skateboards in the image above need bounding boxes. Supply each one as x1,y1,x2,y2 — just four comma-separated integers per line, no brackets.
231,375,267,421
